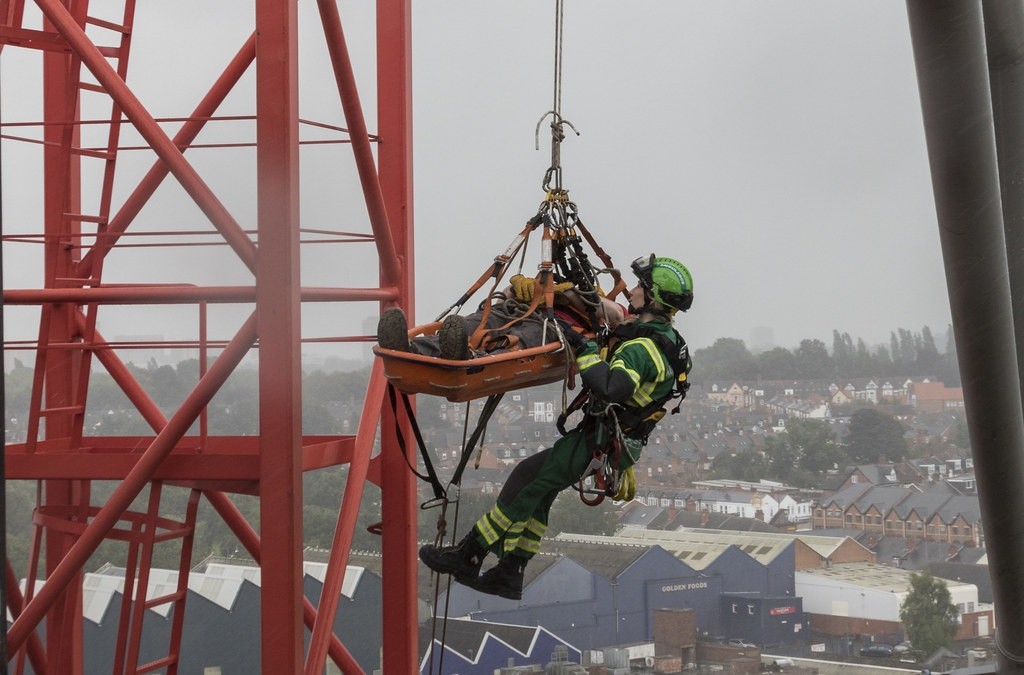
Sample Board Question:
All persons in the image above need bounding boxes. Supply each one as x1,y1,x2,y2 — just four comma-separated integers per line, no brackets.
378,274,628,375
419,253,694,600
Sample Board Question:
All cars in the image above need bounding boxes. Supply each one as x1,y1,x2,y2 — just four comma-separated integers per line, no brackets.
728,638,757,648
893,641,912,656
859,643,893,657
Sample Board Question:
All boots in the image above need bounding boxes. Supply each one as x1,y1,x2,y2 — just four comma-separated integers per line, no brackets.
419,527,487,576
454,552,529,601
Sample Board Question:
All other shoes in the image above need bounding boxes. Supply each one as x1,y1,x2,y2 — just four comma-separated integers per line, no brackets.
438,314,468,360
377,307,410,352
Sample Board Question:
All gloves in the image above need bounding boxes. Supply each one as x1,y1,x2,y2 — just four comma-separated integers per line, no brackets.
510,274,537,302
540,282,574,304
564,327,597,356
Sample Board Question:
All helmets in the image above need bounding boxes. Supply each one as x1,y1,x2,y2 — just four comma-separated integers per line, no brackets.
633,257,694,313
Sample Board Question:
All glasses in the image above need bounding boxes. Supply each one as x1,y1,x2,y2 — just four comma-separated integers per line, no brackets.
631,253,655,284
